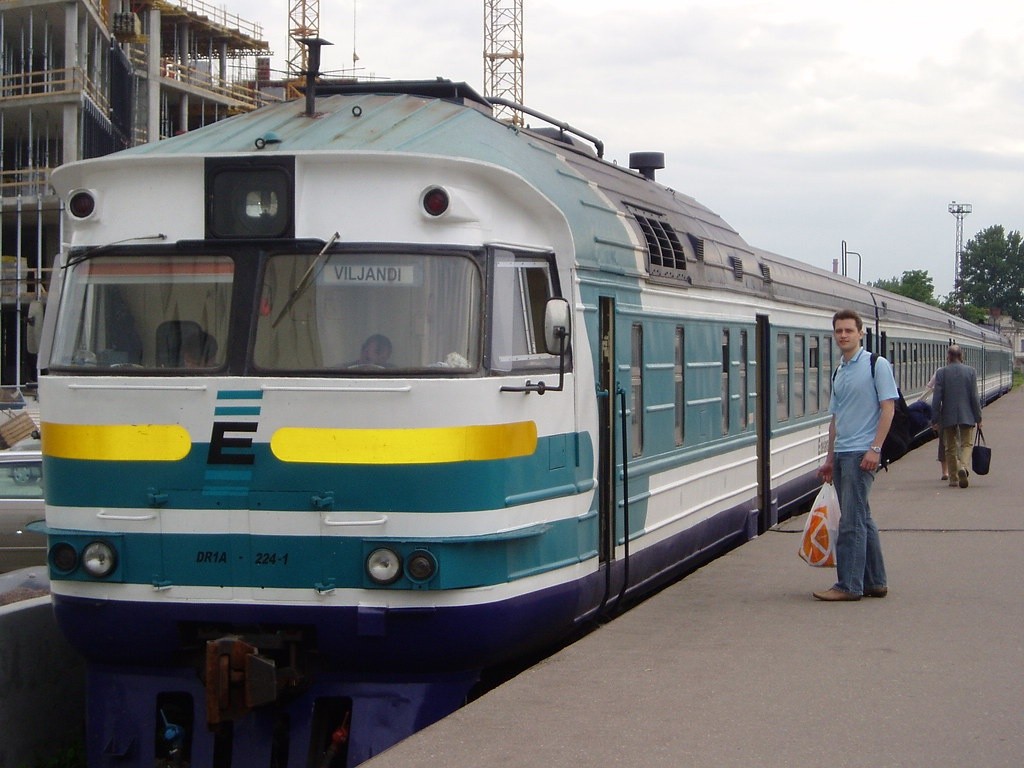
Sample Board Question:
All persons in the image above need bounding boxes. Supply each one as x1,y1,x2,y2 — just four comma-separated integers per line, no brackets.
181,334,219,370
355,335,397,369
813,311,900,600
919,345,983,488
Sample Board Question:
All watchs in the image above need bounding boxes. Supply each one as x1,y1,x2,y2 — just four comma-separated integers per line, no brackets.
870,446,882,453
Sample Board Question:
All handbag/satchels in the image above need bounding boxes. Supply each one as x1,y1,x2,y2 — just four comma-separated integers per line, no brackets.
798,481,840,568
971,426,993,475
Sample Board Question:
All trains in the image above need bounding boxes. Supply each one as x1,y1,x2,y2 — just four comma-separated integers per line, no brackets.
28,33,1017,683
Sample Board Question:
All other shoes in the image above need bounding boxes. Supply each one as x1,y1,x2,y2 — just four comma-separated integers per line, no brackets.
948,476,958,486
941,474,949,481
958,467,969,489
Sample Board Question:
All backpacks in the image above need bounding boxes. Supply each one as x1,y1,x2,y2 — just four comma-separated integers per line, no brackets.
832,352,912,465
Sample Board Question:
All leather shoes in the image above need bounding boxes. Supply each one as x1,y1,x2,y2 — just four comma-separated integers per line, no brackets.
863,589,888,599
813,588,862,601
